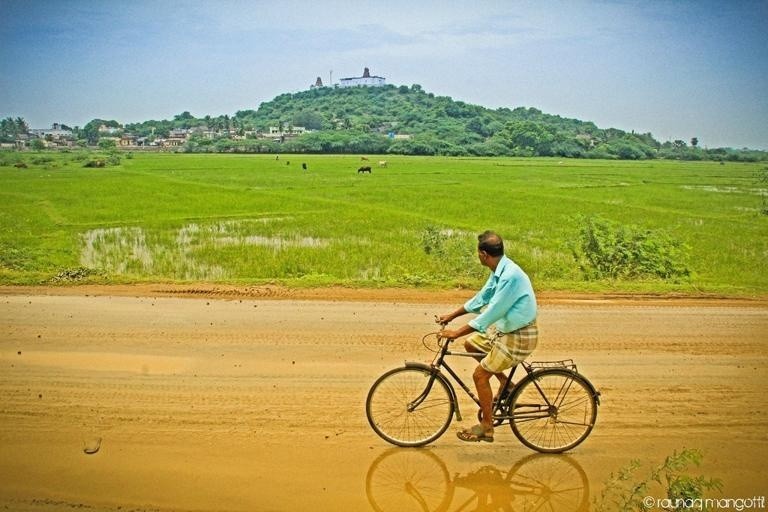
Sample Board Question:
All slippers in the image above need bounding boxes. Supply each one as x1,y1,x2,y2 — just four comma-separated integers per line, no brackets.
456,424,494,443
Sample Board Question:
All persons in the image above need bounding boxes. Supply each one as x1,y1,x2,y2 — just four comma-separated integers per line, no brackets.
435,227,539,443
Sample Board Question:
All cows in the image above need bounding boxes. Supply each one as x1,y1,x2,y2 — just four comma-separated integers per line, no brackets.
361,156,369,161
358,166,371,174
379,160,387,167
13,163,27,168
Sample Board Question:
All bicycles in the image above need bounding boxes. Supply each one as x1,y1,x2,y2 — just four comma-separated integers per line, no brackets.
365,315,601,454
